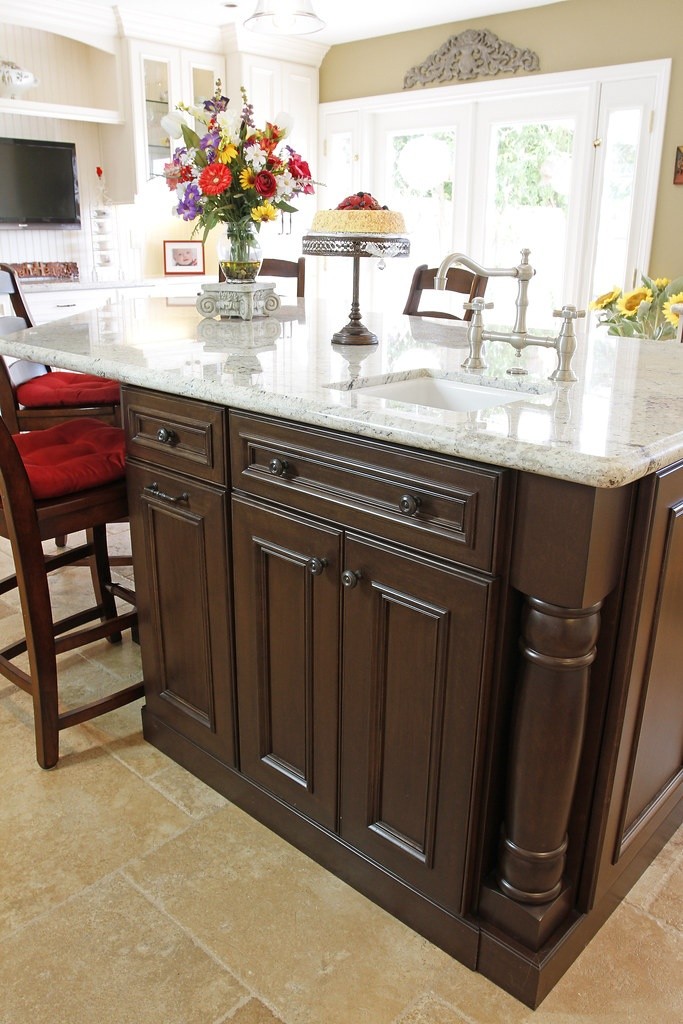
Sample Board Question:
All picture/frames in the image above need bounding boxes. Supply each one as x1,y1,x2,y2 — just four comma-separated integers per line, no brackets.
164,240,205,275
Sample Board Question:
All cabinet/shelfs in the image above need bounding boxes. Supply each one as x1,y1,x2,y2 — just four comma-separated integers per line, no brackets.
119,384,683,1010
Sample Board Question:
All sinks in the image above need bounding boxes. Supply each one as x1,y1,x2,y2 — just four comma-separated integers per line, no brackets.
321,368,555,414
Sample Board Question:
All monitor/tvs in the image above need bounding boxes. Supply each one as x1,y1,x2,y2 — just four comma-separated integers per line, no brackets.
0,137,82,231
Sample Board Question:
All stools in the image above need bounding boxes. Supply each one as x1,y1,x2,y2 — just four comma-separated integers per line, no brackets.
0,263,145,769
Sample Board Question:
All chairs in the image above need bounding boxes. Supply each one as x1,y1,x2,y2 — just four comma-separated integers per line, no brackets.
403,264,488,321
219,257,305,297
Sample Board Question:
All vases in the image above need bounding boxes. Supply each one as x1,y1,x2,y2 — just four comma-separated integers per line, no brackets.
219,221,263,283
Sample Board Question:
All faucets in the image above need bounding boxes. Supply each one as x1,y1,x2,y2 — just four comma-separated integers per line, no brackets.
433,247,536,358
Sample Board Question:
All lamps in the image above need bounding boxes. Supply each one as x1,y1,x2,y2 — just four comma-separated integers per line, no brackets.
244,0,326,34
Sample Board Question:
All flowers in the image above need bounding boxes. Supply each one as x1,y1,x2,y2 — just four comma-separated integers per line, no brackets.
148,79,326,262
591,274,683,341
